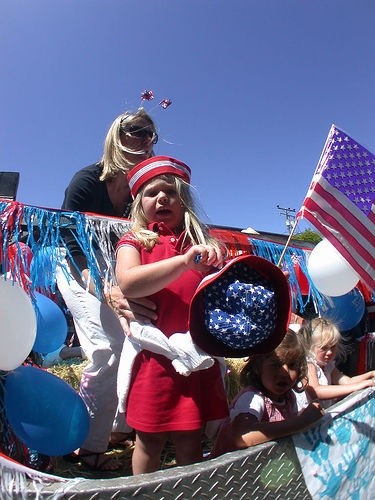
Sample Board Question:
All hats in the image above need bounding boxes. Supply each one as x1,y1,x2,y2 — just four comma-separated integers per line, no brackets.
126,156,191,196
191,256,292,358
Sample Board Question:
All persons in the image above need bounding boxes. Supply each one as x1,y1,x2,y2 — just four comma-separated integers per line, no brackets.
114,155,228,500
49,111,159,473
291,319,375,411
210,329,322,468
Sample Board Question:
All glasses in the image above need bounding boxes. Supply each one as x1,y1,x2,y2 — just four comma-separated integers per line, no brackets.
120,125,160,144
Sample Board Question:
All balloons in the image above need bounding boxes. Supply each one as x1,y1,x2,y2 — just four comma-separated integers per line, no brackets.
307,236,363,298
31,288,67,356
4,366,92,457
317,287,366,330
0,275,38,372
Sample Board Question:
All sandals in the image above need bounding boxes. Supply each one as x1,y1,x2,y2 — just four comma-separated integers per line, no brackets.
109,438,136,448
63,452,122,472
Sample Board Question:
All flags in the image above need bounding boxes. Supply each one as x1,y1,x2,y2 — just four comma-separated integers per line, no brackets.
298,124,374,297
